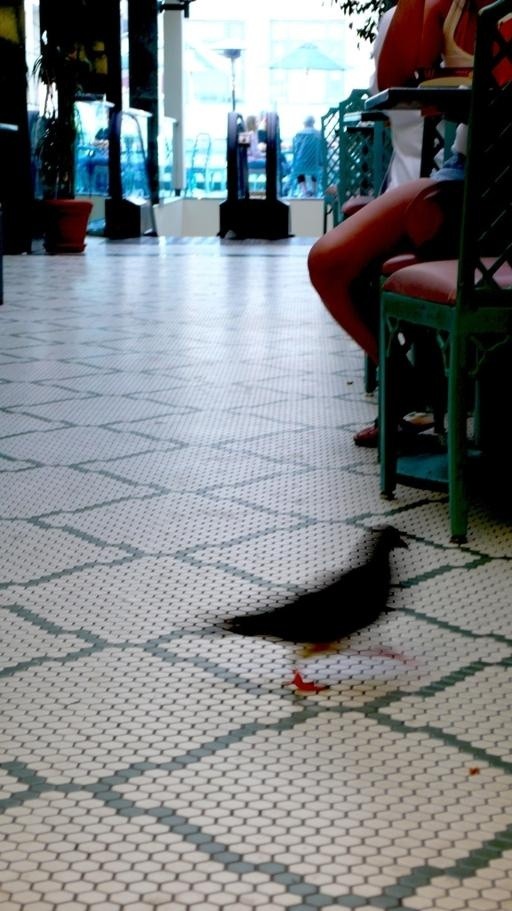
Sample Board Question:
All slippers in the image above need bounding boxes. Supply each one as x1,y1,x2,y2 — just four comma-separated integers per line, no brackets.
354,417,435,447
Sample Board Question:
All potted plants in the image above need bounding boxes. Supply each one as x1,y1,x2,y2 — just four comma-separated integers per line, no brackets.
31,24,95,254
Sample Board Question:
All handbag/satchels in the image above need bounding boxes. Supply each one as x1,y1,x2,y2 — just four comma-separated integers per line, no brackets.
369,0,426,96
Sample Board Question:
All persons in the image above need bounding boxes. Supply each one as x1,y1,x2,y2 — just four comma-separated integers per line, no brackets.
244,114,272,161
349,1,462,198
78,124,116,195
293,110,333,199
310,66,511,456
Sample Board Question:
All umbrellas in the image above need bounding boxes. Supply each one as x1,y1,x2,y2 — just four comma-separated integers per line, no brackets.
269,43,346,110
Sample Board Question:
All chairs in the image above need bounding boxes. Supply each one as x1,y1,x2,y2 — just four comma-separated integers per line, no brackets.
320,1,512,544
76,116,324,198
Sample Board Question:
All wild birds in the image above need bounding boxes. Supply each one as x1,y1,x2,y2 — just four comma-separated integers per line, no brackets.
219,524,416,691
